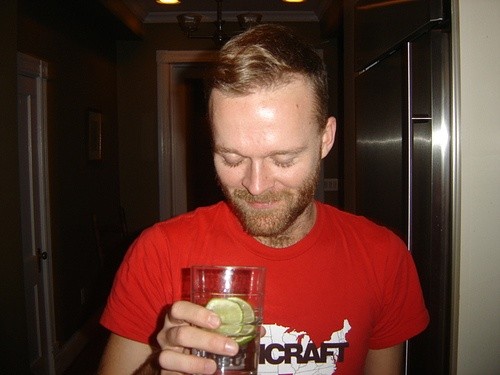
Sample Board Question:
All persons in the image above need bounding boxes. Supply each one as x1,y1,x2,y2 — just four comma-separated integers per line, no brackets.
94,27,430,375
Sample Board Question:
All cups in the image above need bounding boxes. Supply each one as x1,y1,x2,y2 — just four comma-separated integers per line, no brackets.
189,265,264,375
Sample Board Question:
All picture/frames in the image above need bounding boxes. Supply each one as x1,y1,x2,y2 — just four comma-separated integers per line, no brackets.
85,108,104,164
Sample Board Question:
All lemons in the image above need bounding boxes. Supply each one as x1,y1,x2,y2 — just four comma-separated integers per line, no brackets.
205,296,256,346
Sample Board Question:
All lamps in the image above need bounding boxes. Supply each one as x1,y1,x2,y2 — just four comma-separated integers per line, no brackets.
177,0,263,43
